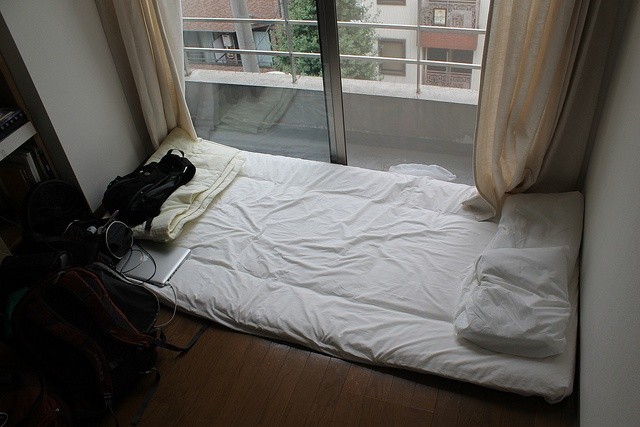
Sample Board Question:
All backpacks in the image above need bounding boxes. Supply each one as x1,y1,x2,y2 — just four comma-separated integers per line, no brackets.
103,148,196,229
1,265,209,427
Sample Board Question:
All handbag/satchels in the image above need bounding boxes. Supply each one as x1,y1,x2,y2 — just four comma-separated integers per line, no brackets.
64,220,134,259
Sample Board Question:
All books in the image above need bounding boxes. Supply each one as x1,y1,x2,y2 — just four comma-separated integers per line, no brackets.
0,142,58,196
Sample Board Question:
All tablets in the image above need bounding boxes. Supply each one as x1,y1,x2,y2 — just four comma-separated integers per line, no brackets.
115,239,191,287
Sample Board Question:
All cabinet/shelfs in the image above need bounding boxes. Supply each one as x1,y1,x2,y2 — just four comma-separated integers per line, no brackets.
0,13,93,260
